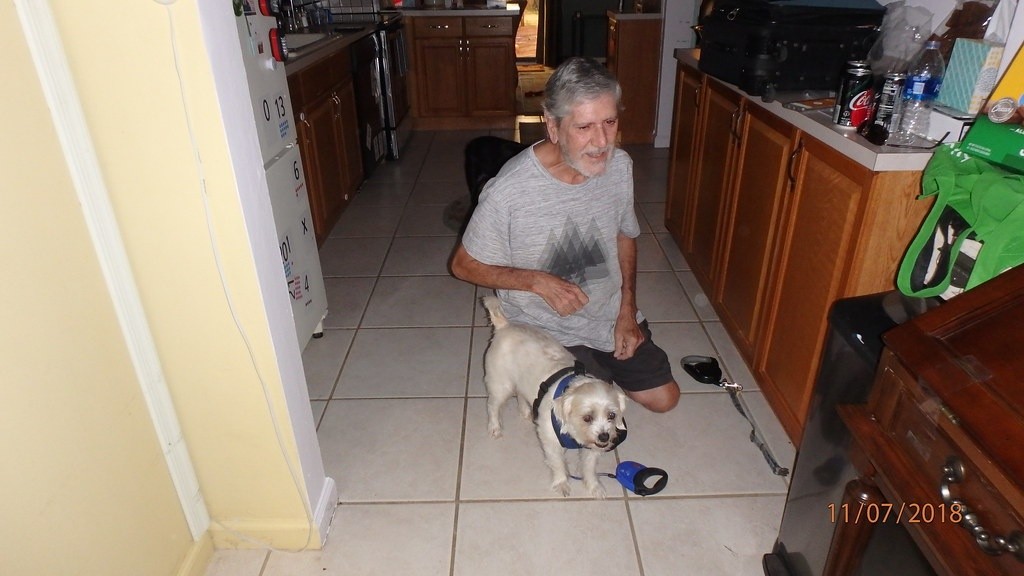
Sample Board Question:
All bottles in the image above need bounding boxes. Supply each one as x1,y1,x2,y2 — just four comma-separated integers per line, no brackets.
892,40,945,148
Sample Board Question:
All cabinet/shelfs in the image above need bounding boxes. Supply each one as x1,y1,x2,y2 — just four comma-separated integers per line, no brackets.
665,61,938,452
403,16,515,130
761,265,1024,576
286,45,366,248
609,18,662,144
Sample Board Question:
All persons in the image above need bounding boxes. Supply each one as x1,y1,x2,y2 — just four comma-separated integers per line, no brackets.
449,55,681,452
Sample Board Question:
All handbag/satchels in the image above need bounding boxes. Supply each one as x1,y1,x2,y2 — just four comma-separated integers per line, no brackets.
896,139,1024,297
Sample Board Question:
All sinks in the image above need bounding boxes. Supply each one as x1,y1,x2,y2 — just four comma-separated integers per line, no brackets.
284,29,346,63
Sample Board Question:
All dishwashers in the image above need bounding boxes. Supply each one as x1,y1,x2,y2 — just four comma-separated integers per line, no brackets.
379,22,417,160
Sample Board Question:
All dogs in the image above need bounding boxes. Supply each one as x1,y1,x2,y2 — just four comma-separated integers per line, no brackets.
482,296,627,501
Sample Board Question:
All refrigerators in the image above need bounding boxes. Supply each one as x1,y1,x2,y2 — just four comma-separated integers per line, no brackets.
233,0,330,355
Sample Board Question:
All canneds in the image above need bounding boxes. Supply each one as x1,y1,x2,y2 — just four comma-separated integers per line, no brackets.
833,60,907,135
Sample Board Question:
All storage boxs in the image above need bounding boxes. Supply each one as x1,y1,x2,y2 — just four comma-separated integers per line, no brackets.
934,38,1006,113
926,104,974,146
